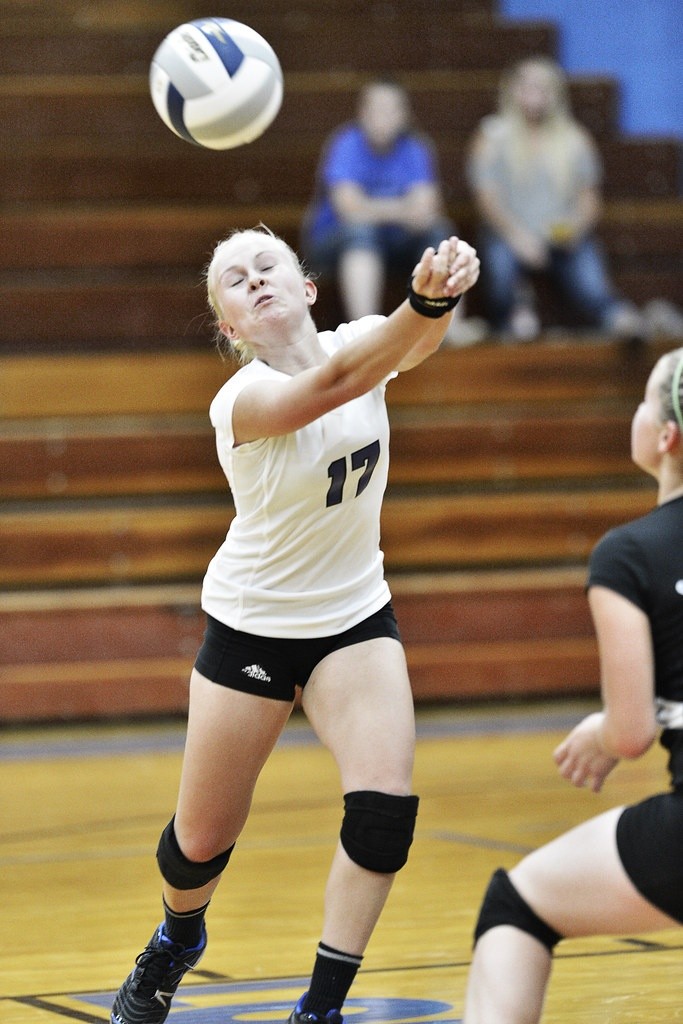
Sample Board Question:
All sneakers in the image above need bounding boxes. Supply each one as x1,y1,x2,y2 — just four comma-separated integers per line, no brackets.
287,992,346,1024
109,916,208,1023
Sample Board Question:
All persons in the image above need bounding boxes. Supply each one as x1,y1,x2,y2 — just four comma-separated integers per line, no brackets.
463,44,643,349
300,69,466,362
104,221,479,1024
461,347,683,1024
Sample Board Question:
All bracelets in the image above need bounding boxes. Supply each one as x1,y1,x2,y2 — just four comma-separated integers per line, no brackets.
410,278,461,320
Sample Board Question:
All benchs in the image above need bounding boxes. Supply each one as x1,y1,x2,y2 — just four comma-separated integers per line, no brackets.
1,1,683,723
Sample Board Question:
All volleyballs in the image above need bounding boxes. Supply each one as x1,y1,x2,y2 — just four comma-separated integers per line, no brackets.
146,16,286,151
